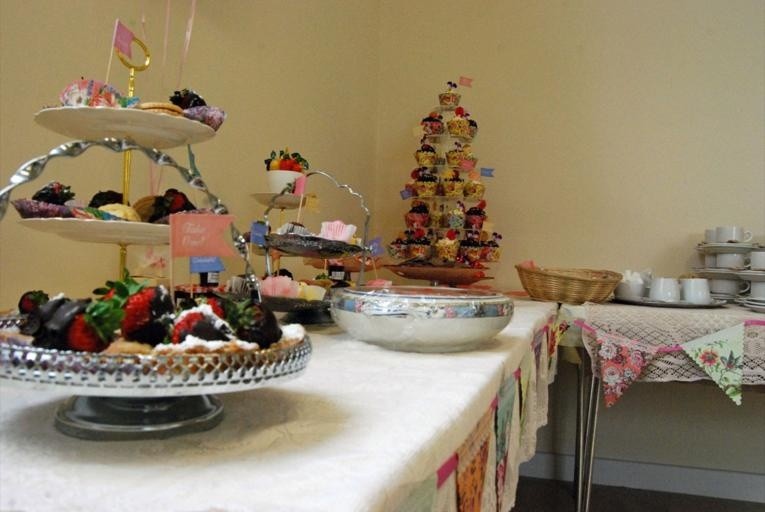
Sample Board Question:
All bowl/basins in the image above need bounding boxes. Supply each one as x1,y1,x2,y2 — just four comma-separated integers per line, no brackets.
330,285,514,354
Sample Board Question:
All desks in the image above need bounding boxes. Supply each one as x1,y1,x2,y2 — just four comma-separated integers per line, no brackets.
0,299,765,512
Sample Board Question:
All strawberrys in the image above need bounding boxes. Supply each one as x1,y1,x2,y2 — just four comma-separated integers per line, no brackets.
61,287,282,353
268,147,303,171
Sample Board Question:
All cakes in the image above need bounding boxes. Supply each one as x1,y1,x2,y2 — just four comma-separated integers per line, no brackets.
388,80,503,265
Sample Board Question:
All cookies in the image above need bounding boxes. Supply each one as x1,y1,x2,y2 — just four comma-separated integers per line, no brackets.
138,102,185,117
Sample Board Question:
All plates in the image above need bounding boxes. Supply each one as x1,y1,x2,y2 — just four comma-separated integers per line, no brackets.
692,268,765,279
710,294,765,314
612,300,728,308
694,243,759,253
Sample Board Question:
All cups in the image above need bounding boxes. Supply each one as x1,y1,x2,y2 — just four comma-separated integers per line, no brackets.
649,278,681,302
751,280,765,298
750,252,765,270
614,282,647,302
704,253,751,268
704,225,753,243
681,278,711,304
711,279,751,294
